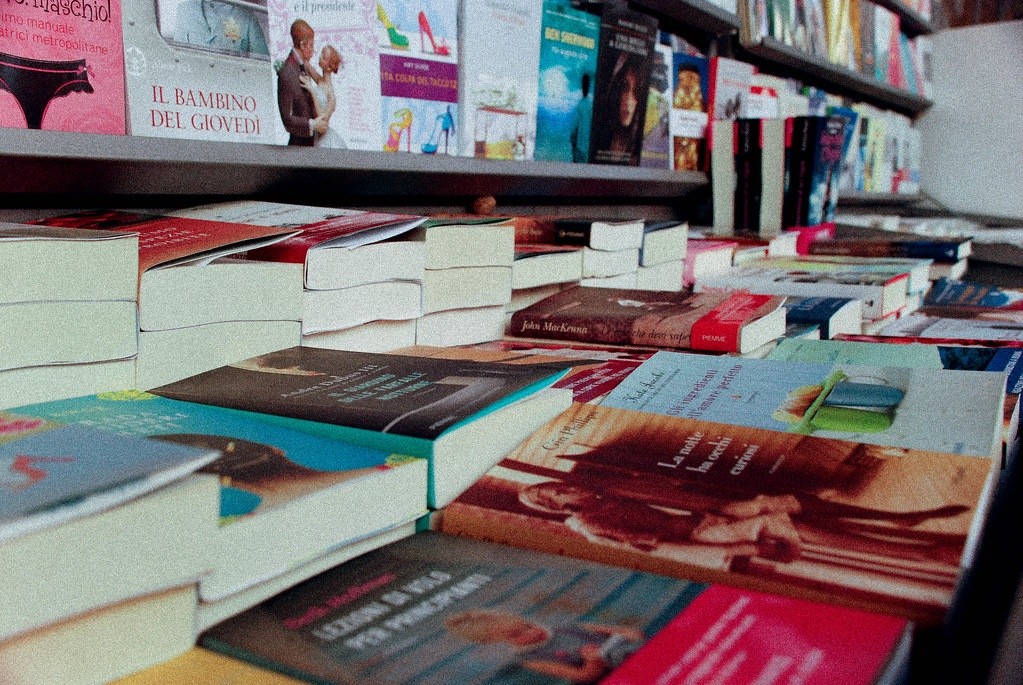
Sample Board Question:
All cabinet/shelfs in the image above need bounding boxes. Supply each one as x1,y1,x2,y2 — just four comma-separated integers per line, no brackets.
0,0,1023,685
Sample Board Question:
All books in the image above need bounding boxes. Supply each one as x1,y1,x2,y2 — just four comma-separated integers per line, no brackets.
0,0,1023,685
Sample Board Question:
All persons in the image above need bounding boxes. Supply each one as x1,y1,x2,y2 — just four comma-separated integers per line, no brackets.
299,44,348,150
446,609,644,682
149,434,381,514
569,72,595,162
598,50,649,165
277,19,328,147
518,478,970,557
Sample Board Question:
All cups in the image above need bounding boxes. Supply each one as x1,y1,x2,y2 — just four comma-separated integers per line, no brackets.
810,405,891,435
824,374,905,407
